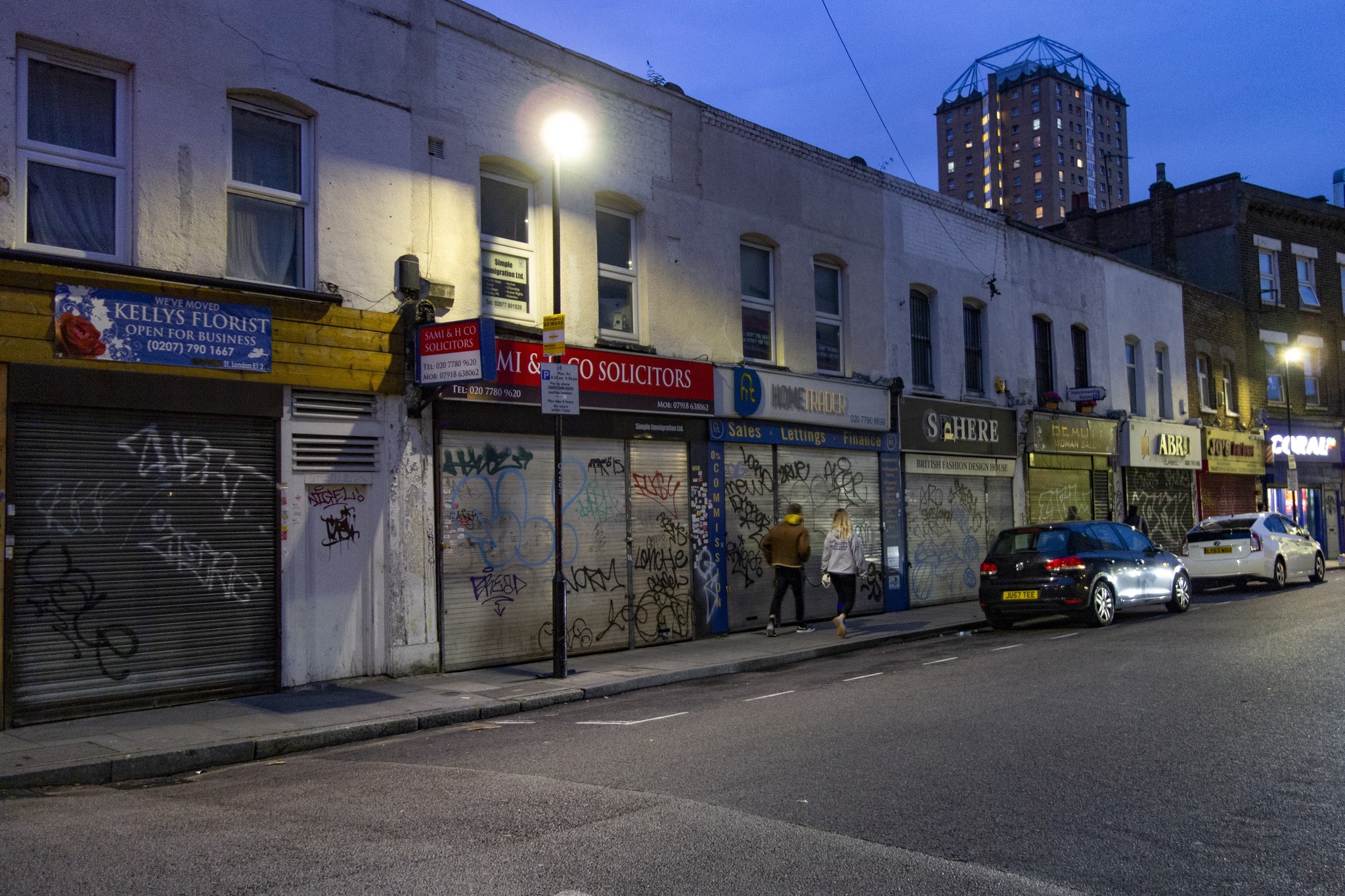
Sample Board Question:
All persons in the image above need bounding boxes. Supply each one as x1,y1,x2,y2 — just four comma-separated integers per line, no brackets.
759,502,816,637
821,508,869,637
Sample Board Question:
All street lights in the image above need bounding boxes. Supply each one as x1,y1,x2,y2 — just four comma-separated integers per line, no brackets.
1280,346,1303,526
536,108,591,675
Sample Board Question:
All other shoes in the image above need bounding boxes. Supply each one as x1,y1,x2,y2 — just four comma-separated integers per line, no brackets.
767,623,777,637
796,625,815,633
833,616,846,638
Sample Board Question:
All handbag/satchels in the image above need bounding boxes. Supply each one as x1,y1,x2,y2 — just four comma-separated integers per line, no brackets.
822,573,832,588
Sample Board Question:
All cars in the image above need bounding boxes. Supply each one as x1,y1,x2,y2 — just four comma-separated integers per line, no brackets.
979,519,1190,628
1179,512,1326,592
